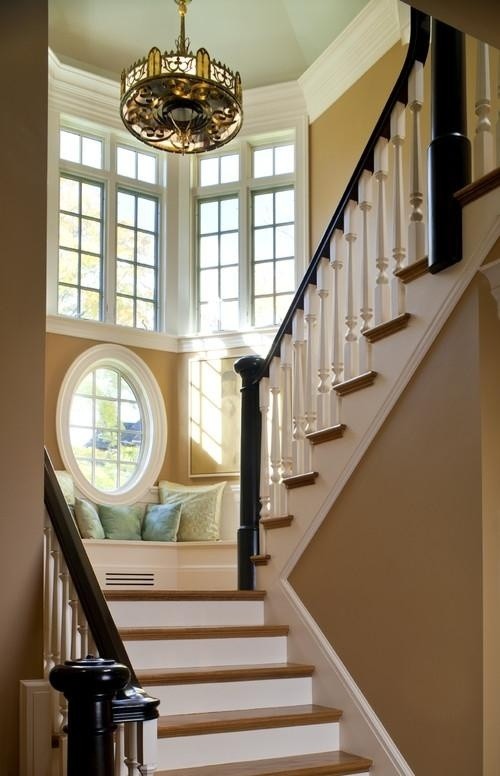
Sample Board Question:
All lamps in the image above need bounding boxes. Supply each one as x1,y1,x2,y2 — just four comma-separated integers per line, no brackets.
116,3,244,156
